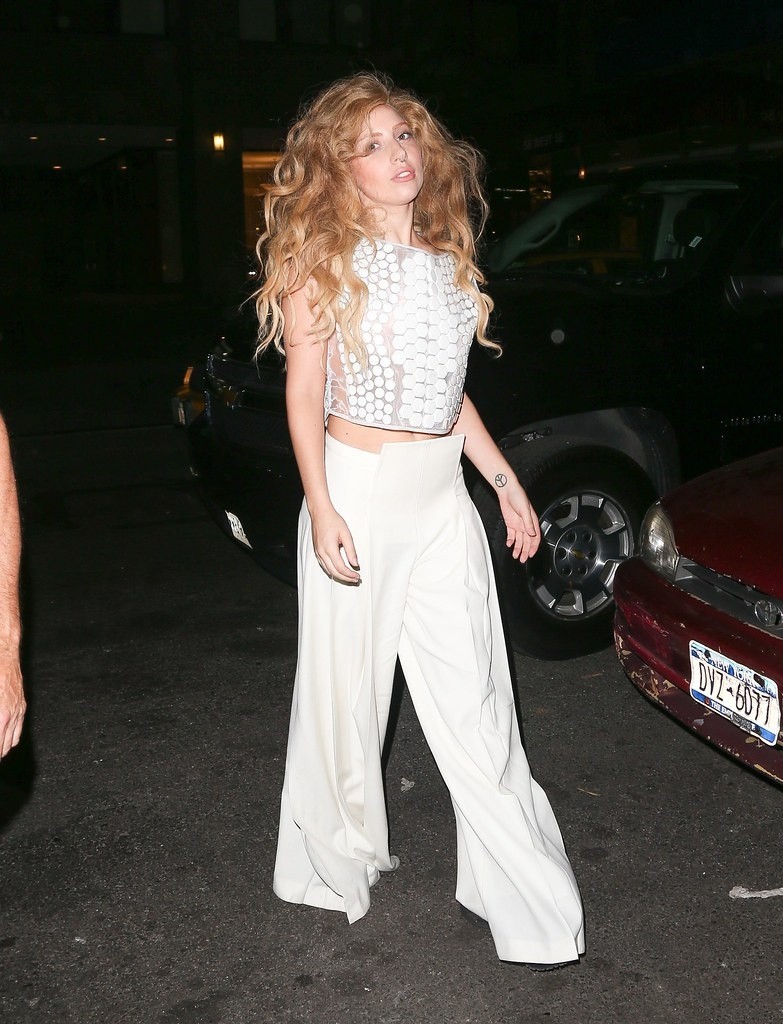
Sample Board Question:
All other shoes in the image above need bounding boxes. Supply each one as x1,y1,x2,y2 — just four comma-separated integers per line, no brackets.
524,957,579,972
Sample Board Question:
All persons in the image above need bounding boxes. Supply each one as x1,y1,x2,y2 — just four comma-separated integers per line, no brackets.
238,69,588,977
0,412,30,756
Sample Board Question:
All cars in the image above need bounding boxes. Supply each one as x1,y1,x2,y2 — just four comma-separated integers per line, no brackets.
168,150,782,662
606,445,783,795
